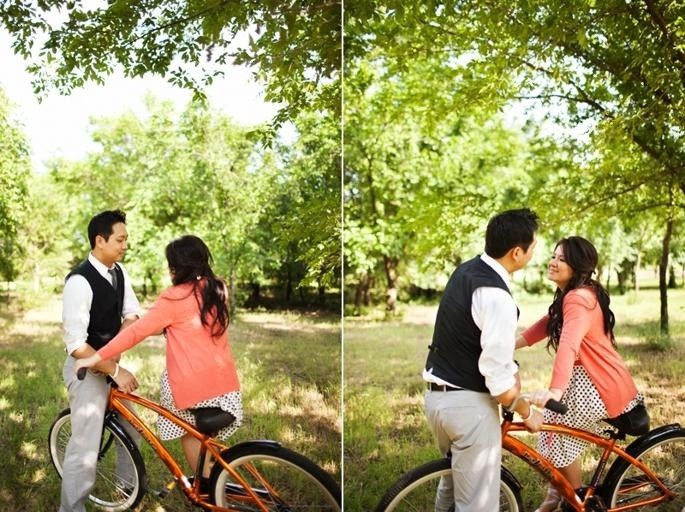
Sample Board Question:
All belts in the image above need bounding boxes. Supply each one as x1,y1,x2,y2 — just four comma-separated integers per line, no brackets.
427,382,462,390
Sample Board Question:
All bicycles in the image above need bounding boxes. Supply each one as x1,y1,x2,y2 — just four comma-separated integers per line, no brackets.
46,366,342,511
377,344,685,512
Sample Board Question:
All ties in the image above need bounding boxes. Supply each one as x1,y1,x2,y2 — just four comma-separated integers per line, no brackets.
108,270,118,290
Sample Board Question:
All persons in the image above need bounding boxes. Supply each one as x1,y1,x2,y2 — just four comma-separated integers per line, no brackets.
54,208,152,512
418,207,538,511
72,234,244,498
516,235,645,511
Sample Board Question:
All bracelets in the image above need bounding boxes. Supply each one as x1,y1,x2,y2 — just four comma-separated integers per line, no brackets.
110,362,119,377
522,405,533,420
